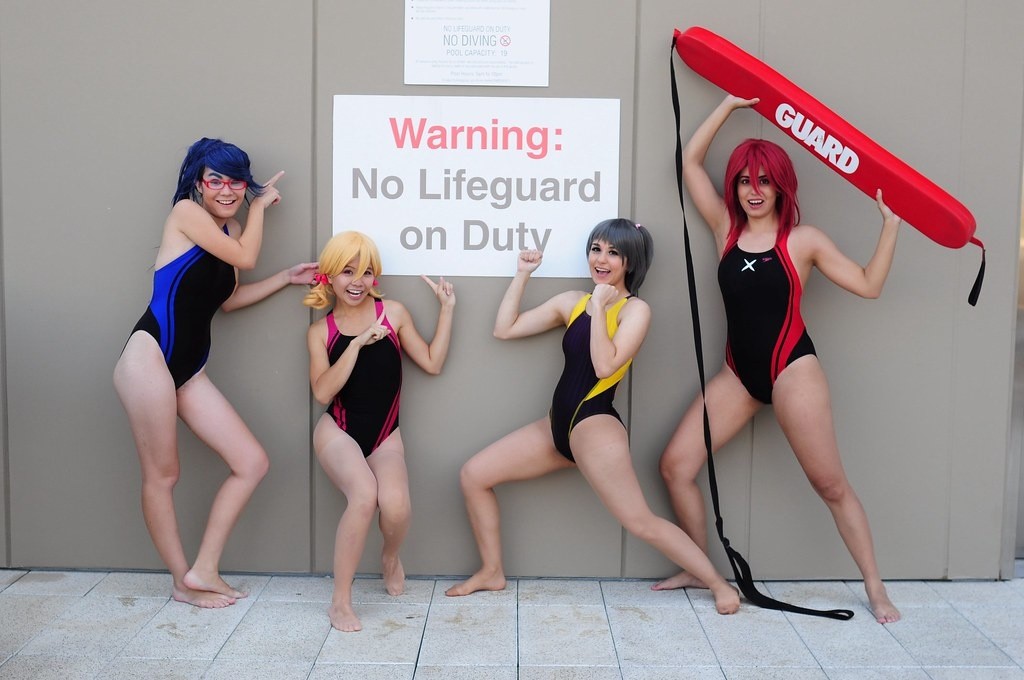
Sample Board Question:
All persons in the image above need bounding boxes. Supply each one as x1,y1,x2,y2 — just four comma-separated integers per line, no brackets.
307,230,457,634
649,92,902,625
444,218,741,618
110,135,325,610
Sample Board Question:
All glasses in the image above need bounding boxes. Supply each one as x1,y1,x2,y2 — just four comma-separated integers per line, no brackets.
200,178,247,190
